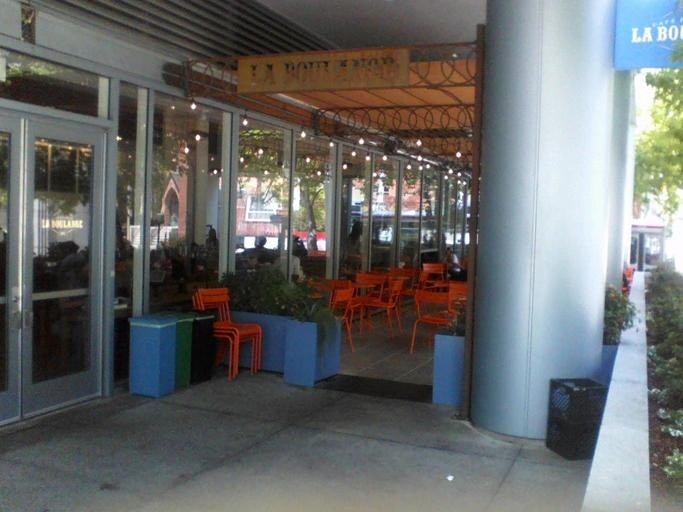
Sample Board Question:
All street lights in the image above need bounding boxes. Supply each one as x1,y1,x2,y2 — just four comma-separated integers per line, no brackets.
153,212,163,250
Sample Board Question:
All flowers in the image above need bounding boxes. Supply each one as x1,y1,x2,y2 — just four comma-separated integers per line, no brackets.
604,282,642,344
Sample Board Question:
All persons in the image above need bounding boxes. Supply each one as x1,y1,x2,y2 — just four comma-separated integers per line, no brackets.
244,236,275,262
273,241,300,282
291,232,307,253
343,220,364,262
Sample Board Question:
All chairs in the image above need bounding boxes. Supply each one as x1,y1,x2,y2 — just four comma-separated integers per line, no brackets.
304,262,468,354
191,287,263,383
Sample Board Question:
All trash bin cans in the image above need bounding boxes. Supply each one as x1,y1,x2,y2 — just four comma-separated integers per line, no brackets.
545,377,611,461
127,312,214,395
